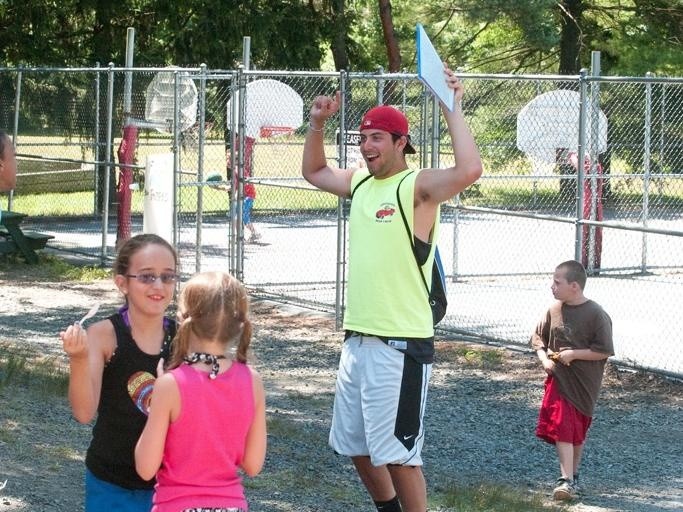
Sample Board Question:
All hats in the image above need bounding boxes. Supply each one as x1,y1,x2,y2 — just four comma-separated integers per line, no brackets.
358,105,416,155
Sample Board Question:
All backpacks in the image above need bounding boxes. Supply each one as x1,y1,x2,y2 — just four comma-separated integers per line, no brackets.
350,170,448,328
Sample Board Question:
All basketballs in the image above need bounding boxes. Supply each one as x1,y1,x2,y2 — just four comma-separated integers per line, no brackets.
207,171,222,186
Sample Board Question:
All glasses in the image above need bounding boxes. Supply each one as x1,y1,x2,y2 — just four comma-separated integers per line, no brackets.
124,272,178,286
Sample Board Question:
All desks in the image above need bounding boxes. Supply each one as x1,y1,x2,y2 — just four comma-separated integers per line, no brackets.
1,209,38,265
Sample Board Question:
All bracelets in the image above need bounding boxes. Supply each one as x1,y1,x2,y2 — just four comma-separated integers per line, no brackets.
307,122,325,132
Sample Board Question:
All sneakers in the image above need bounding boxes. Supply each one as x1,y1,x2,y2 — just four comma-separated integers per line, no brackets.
552,473,582,501
246,230,258,243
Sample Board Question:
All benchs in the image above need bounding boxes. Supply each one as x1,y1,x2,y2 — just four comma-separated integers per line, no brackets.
1,230,54,249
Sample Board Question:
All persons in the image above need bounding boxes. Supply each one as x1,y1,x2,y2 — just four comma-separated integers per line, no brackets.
60,234,181,510
132,267,271,511
527,256,615,503
0,125,18,224
213,149,262,244
303,71,483,512
